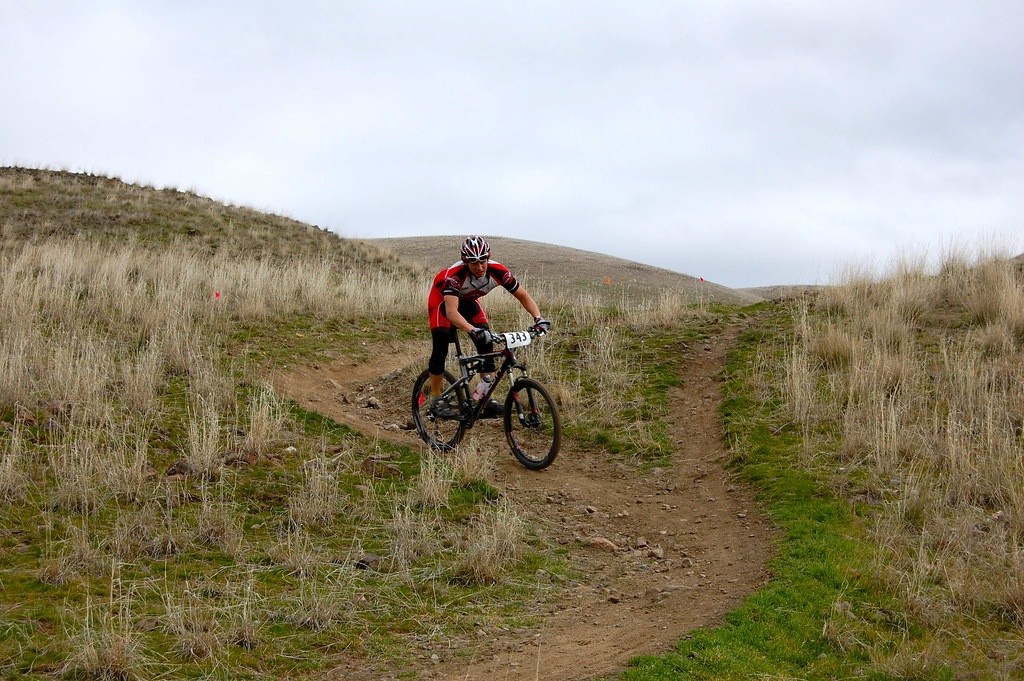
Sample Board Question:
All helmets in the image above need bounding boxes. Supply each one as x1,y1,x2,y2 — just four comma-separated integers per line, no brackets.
461,236,490,261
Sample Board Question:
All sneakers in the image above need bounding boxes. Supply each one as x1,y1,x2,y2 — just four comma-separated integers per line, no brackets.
484,399,505,415
430,399,456,418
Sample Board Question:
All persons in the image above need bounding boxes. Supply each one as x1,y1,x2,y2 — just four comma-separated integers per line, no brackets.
427,235,549,419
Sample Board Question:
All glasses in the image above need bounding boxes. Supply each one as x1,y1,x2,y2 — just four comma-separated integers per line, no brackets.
466,252,489,264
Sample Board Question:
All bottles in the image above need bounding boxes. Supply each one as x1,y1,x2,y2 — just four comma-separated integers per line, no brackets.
473,374,492,400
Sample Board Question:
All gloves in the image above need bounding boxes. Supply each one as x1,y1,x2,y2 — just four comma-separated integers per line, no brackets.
529,316,547,337
471,328,492,344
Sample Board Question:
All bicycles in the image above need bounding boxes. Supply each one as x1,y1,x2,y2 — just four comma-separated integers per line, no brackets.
410,328,563,471
